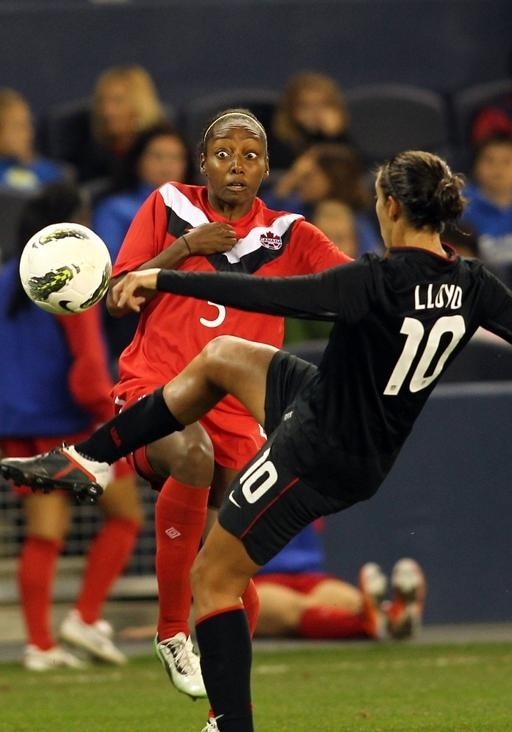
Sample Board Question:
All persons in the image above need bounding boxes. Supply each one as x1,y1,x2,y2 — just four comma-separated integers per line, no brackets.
69,62,164,187
1,88,69,251
1,184,135,671
239,516,430,640
90,130,187,341
99,106,357,732
272,72,511,283
0,146,512,732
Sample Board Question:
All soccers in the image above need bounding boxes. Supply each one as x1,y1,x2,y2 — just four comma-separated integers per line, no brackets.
19,223,114,315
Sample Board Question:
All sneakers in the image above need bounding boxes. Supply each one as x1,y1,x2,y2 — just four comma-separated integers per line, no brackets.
358,557,426,642
154,632,209,701
58,608,128,665
0,440,112,506
23,643,90,672
